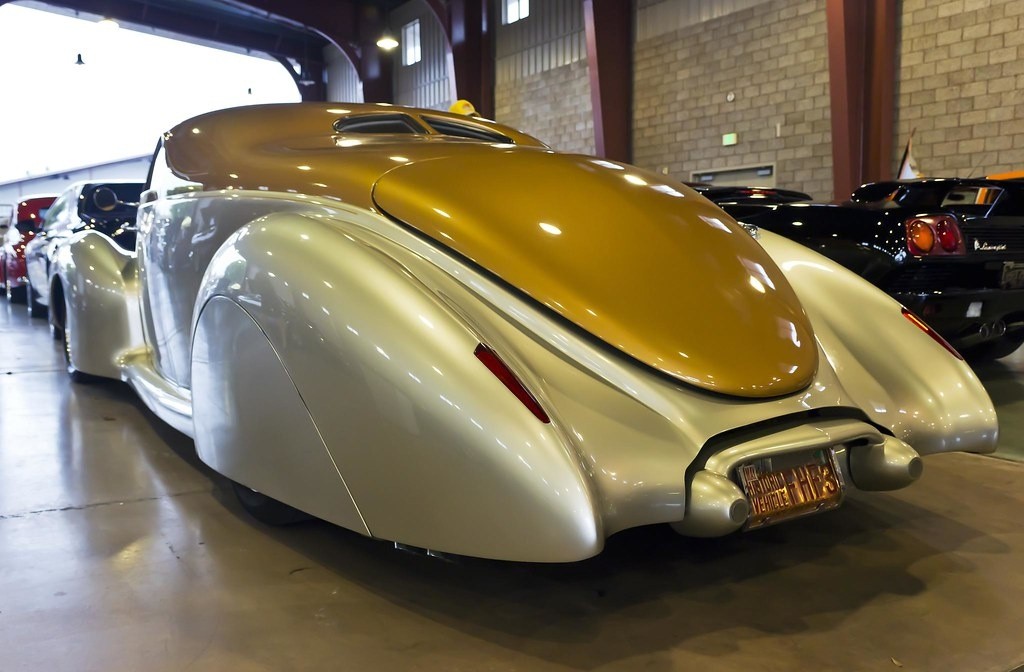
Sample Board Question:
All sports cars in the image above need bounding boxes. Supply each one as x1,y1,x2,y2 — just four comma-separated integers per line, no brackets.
46,103,1000,568
678,171,1024,364
14,180,145,340
0,193,59,303
